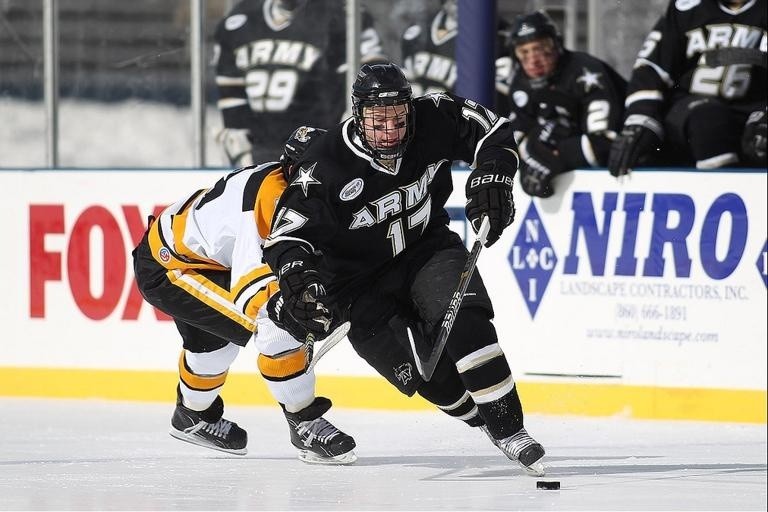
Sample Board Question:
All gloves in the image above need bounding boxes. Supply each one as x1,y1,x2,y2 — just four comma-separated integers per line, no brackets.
746,107,767,168
519,152,568,197
220,128,256,170
267,293,325,343
608,126,653,175
265,245,334,334
466,150,522,247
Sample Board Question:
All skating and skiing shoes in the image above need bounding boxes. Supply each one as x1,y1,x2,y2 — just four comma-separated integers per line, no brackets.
166,384,250,457
494,428,547,476
278,397,357,465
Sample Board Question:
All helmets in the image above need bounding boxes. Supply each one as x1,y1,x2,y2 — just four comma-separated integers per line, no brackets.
351,64,416,161
510,12,560,87
281,126,327,180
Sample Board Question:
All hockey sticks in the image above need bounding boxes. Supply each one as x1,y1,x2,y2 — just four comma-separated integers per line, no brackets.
406,215,491,383
303,320,352,376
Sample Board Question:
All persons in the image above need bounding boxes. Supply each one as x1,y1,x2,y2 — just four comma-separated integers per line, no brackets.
401,0,520,117
263,59,546,477
132,125,356,464
610,0,766,178
507,9,634,199
212,0,383,171
